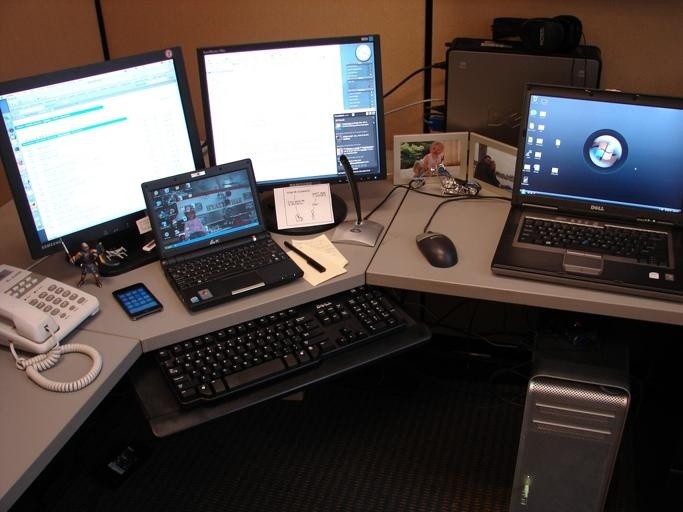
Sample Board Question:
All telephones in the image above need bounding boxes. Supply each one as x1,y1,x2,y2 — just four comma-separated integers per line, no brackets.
0,263,102,392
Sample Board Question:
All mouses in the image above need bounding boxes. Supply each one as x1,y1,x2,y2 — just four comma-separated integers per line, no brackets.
416,230,457,268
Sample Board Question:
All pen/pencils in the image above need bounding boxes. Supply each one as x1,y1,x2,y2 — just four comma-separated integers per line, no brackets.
284,241,326,273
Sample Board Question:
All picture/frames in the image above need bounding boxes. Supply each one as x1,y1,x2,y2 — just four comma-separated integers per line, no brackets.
469,132,518,198
393,132,469,186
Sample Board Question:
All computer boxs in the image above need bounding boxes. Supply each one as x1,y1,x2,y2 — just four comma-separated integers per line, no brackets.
442,37,603,148
504,323,632,511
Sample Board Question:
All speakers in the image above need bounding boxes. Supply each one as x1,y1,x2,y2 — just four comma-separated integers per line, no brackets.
492,17,545,49
548,14,583,49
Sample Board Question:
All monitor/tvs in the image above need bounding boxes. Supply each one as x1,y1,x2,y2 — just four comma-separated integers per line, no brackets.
1,46,206,278
197,35,386,236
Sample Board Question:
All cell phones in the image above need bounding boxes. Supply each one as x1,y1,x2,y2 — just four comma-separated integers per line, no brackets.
113,283,163,321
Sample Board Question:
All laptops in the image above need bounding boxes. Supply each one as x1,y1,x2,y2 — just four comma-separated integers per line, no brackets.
490,83,683,304
141,158,303,313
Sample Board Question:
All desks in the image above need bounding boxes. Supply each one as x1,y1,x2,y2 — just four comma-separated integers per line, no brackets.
0,149,681,511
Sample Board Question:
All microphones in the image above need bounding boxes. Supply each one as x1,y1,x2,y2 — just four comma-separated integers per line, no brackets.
331,155,384,248
157,283,408,409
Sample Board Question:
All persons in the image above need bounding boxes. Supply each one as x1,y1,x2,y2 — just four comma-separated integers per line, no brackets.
475,155,510,189
183,205,207,240
412,142,448,177
68,241,103,288
